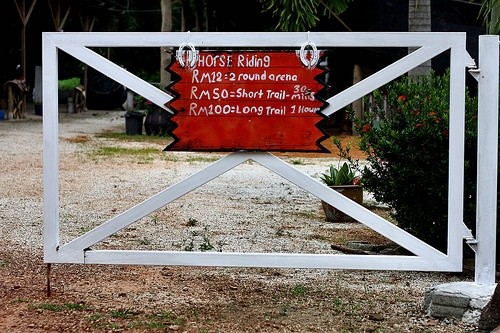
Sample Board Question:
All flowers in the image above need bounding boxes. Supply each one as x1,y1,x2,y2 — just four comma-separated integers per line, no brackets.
136,96,152,105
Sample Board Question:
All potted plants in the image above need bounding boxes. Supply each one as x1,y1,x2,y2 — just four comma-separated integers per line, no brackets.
319,163,364,223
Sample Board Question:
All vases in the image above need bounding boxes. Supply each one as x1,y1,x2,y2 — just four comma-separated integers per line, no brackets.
124,112,146,135
144,103,165,134
